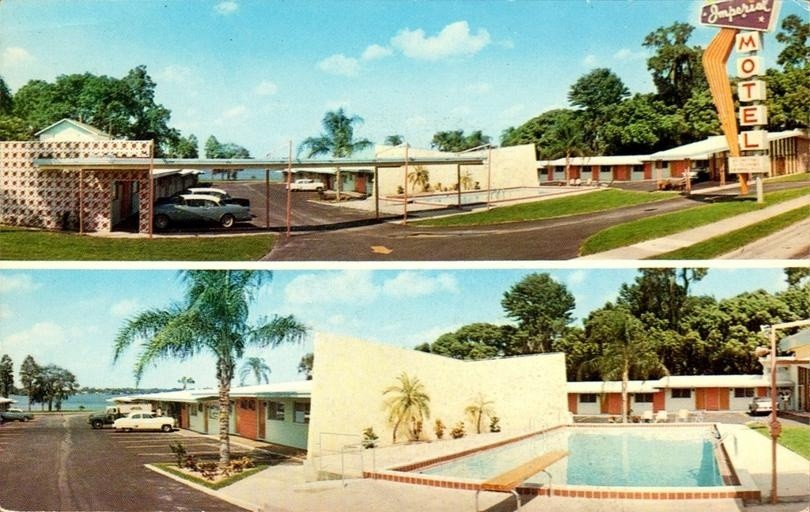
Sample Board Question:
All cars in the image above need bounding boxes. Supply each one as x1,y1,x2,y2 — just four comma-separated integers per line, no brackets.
286,179,328,192
153,194,252,227
0,409,34,423
112,412,177,432
749,396,780,416
185,186,230,201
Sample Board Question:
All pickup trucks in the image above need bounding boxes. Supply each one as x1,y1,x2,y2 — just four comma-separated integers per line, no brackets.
88,407,131,429
